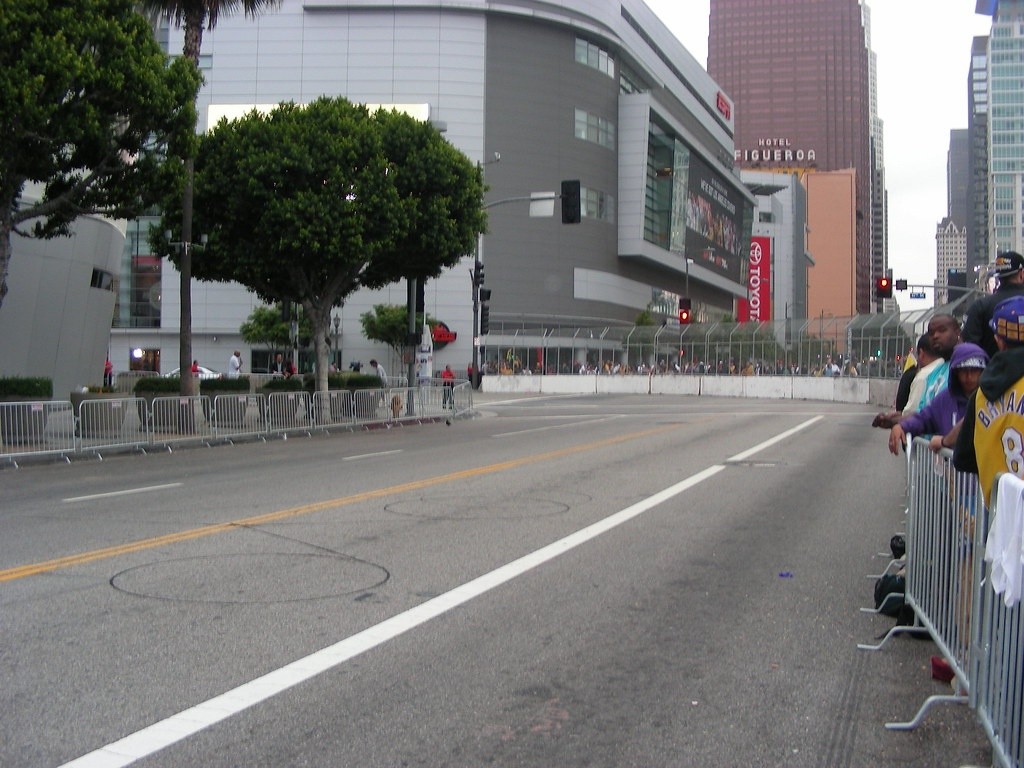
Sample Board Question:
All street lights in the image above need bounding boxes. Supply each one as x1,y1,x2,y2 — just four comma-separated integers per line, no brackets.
685,247,715,297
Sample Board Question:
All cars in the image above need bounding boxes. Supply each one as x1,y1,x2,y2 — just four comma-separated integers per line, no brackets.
161,364,228,380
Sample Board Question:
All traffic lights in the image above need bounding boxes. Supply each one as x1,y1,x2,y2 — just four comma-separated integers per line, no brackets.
474,261,485,286
678,297,691,324
481,303,490,336
480,289,491,301
877,277,893,298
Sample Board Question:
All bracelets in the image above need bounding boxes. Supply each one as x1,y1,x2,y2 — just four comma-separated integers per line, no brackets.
941,436,947,447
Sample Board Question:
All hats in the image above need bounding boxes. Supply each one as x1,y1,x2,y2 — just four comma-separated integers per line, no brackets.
989,296,1024,344
994,252,1024,277
956,355,986,369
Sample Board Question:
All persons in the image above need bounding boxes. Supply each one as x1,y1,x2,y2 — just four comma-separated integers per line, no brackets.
686,190,742,256
192,360,202,377
369,359,387,401
467,356,905,389
281,354,297,381
329,362,337,371
270,354,284,379
229,350,242,379
441,365,455,410
104,361,113,386
871,251,1024,760
